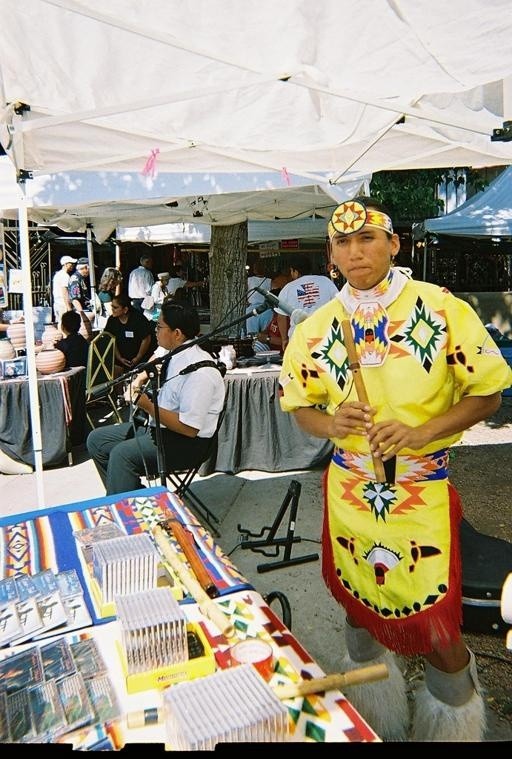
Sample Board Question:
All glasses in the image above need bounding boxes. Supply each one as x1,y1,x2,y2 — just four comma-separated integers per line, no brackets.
156,323,171,330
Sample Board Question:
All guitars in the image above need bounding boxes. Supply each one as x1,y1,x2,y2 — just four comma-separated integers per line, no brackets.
132,379,161,428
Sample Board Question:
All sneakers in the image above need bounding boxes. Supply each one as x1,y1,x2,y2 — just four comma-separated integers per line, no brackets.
117,398,126,412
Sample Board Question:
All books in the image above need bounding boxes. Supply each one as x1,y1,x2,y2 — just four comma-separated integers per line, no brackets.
0,567,122,751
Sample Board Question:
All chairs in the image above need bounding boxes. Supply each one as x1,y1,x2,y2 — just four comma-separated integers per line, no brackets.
86,331,123,430
145,410,226,538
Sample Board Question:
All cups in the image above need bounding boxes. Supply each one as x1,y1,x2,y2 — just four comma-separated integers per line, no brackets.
230,637,273,684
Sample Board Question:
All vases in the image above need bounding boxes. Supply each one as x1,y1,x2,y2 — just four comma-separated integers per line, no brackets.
36,349,67,375
7,321,25,350
76,310,95,340
0,338,16,361
42,322,63,347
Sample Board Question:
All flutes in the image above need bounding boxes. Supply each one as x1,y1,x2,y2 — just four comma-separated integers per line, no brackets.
342,319,386,484
270,663,390,700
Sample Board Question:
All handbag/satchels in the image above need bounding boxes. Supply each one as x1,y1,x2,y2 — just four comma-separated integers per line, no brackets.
140,296,154,311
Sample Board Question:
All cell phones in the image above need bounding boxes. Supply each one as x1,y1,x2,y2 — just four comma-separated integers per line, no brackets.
186,631,203,658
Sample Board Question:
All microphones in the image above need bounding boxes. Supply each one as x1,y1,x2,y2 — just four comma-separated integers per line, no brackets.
254,287,308,325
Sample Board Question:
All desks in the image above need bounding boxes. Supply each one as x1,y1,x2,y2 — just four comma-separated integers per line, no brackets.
0,486,384,751
215,355,335,476
1,365,87,467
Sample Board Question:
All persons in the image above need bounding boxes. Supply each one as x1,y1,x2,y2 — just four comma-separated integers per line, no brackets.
277,195,512,742
246,254,340,358
51,252,227,497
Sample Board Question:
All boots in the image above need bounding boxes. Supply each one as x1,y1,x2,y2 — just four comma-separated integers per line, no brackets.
413,645,487,742
339,616,411,741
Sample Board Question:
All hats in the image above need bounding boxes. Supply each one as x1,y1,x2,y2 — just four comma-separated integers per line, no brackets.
60,256,77,266
158,272,169,279
79,258,89,265
